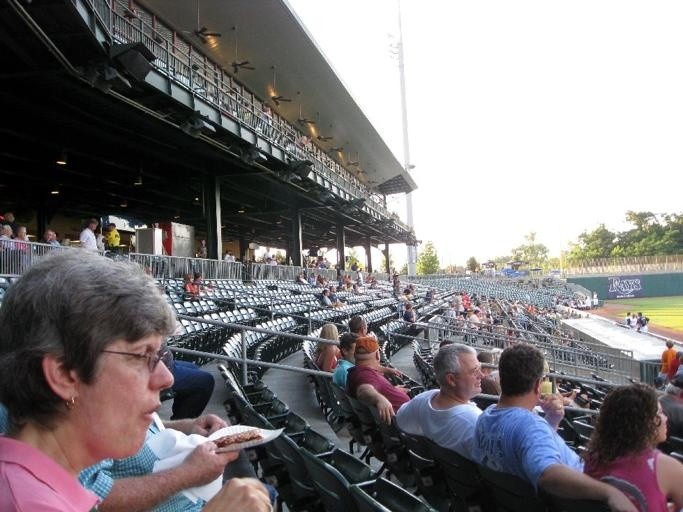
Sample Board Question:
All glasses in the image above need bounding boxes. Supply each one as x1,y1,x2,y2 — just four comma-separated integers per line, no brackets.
473,362,482,376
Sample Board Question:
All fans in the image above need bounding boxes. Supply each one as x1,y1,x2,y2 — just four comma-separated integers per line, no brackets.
179,1,380,187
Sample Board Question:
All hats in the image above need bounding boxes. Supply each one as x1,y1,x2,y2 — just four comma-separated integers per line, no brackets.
355,335,378,355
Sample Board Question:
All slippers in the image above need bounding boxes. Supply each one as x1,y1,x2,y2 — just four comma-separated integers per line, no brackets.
98,347,164,372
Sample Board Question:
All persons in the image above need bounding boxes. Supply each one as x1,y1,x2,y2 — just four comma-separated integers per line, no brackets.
256,105,273,134
300,136,312,146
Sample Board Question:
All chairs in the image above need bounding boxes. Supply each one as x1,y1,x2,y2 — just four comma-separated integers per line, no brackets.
658,436,683,462
154,275,617,512
625,376,641,385
0,274,22,297
